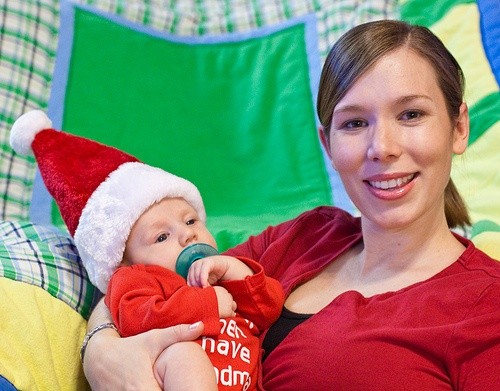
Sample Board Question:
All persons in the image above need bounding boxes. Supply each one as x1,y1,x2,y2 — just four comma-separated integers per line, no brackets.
80,16,499,390
8,108,285,391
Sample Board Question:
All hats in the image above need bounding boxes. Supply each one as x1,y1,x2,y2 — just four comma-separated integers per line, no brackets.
9,110,205,294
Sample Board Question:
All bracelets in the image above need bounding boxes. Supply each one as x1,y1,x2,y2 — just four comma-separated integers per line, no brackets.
80,322,121,364
